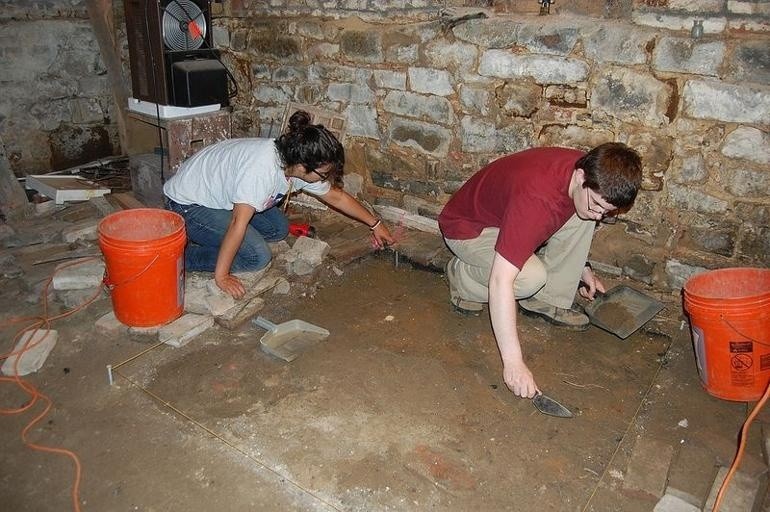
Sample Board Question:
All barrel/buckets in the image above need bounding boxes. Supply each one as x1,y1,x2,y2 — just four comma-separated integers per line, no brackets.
681,265,770,401
95,206,188,329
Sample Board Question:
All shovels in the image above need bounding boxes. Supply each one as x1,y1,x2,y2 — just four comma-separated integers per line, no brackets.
531,392,573,417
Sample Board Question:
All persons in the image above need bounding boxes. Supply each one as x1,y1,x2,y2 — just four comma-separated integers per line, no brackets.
162,111,394,298
436,137,642,398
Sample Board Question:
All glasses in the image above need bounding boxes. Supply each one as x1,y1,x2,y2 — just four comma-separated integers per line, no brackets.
313,168,330,182
587,188,611,218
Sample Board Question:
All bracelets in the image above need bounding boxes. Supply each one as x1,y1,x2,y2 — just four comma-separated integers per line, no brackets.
584,261,592,269
369,219,381,231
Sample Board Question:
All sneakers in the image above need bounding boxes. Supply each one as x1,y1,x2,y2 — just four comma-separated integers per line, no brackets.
444,255,483,317
518,295,589,331
